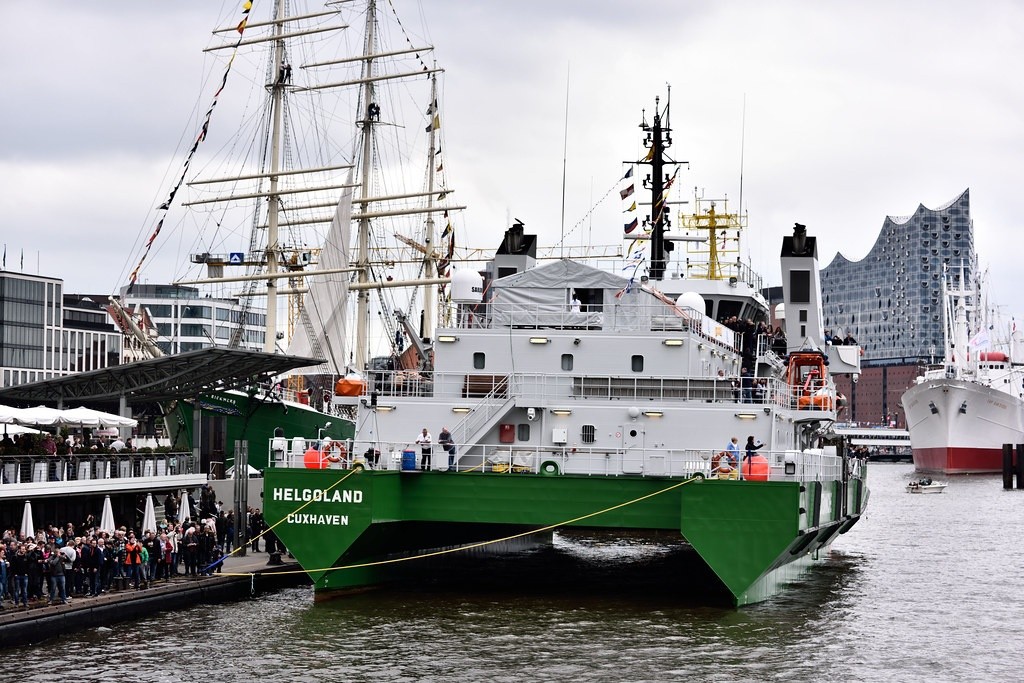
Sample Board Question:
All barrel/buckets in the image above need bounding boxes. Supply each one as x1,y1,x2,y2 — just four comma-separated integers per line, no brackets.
402,449,416,470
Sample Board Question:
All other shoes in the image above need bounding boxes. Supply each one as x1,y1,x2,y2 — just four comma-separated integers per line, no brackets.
257,550,261,552
60,600,68,604
227,551,231,554
191,573,196,576
185,572,189,576
48,601,52,604
66,596,72,600
129,583,134,586
14,603,19,608
24,604,30,607
10,599,15,604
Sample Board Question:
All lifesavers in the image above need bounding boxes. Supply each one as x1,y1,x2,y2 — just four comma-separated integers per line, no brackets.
714,451,737,473
324,441,345,462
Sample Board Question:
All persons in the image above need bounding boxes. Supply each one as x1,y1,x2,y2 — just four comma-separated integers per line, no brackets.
571,294,581,329
0,483,295,609
718,315,786,404
415,427,433,470
438,428,455,468
745,436,766,457
825,328,858,346
727,437,743,480
0,428,132,480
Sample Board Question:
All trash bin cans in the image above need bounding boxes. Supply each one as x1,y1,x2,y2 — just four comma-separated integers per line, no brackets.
402,449,415,470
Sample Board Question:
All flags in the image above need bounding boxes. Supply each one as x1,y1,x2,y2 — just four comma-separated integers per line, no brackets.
3,245,6,267
615,165,644,299
20,250,23,270
127,0,253,295
425,99,454,293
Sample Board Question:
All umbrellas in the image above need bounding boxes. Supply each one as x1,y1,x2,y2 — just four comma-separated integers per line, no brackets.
178,491,191,524
142,493,157,535
0,405,137,442
101,494,115,537
20,501,34,540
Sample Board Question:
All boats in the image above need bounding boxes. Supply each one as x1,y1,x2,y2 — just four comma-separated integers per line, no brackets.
905,480,950,494
257,67,870,608
899,250,1024,478
799,395,832,413
336,376,366,397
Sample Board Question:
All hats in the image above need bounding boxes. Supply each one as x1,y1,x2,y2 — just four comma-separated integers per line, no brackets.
49,535,56,539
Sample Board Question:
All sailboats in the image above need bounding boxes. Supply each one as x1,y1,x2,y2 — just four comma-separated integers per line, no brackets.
100,0,467,475
